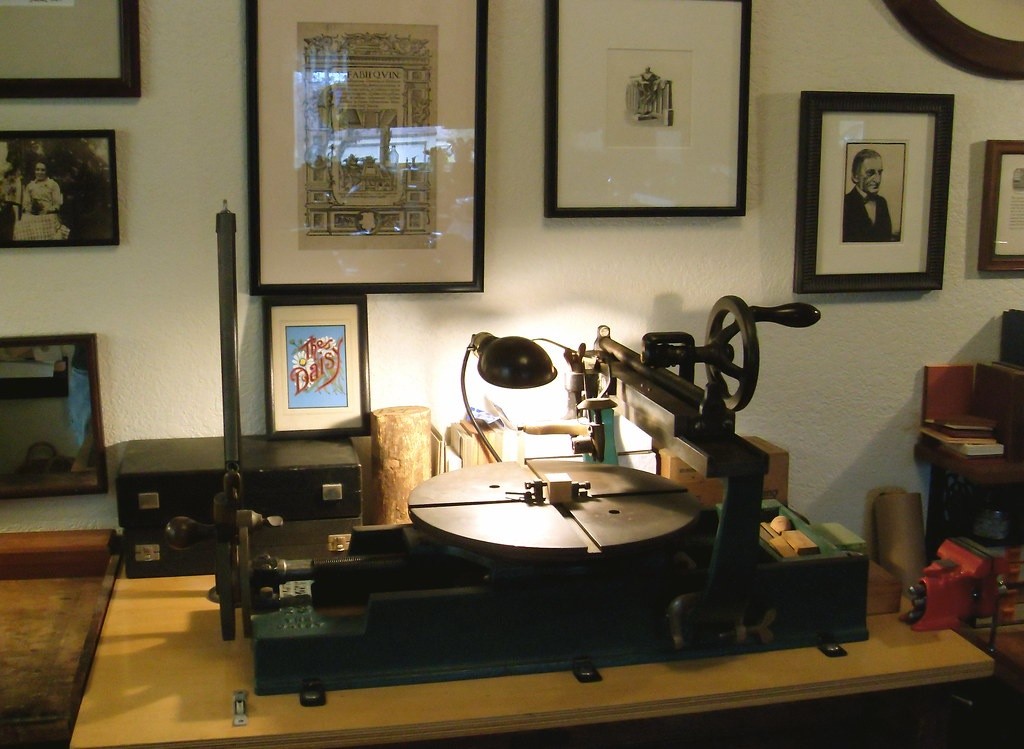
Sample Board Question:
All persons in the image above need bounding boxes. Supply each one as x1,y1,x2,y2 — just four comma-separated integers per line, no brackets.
12,160,71,241
843,149,892,242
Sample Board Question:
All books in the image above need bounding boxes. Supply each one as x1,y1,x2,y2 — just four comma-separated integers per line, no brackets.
918,412,1006,461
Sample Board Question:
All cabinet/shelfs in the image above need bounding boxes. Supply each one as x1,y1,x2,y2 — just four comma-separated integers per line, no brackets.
919,446,1022,694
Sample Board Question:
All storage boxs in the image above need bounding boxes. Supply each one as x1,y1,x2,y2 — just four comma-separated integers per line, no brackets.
123,517,363,580
655,437,789,512
113,434,363,526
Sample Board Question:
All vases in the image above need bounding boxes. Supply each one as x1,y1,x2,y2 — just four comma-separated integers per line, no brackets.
793,89,955,294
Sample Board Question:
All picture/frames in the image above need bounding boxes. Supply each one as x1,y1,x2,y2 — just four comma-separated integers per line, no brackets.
0,333,109,499
0,0,142,98
543,0,753,217
262,293,372,439
0,128,122,247
246,0,487,296
881,0,1024,83
977,139,1024,273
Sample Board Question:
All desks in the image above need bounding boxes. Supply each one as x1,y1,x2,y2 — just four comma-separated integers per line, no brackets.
66,574,996,749
